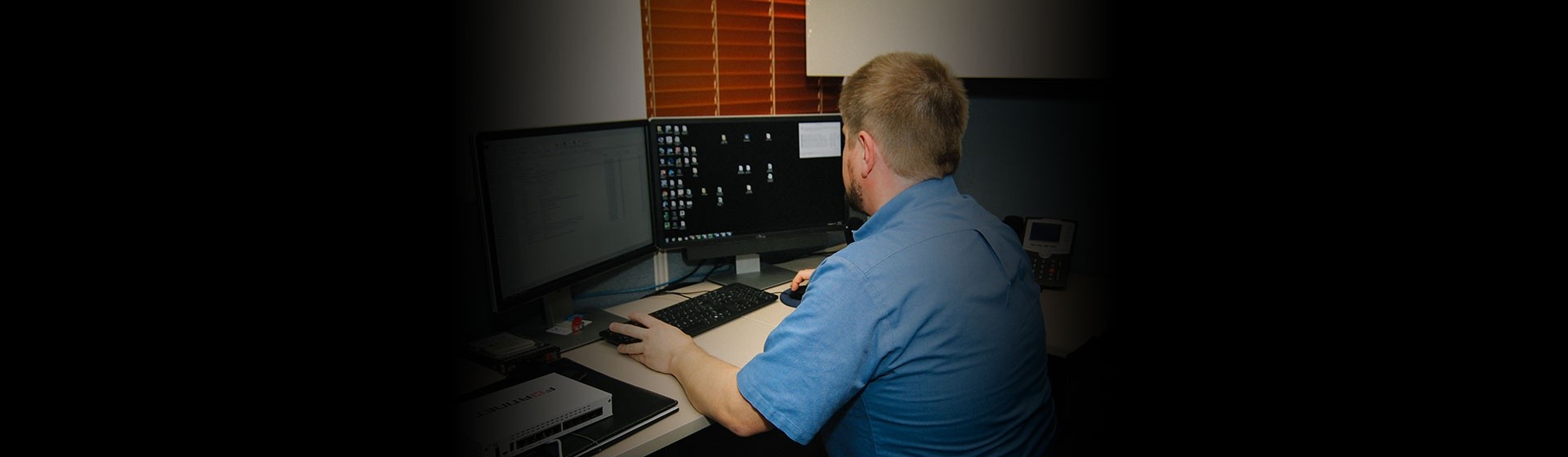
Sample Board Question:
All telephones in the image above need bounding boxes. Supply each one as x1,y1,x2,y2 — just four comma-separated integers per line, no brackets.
1002,216,1077,291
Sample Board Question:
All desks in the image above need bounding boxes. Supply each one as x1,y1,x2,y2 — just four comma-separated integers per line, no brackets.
463,239,1107,457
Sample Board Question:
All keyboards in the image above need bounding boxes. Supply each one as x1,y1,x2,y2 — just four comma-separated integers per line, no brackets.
599,283,779,346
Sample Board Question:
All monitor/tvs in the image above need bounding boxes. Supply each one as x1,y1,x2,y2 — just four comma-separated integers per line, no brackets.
470,113,853,352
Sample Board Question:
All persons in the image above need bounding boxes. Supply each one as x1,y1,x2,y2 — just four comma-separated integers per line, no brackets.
609,53,1060,457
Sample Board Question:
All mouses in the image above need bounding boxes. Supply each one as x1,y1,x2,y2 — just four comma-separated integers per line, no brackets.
789,285,807,300
